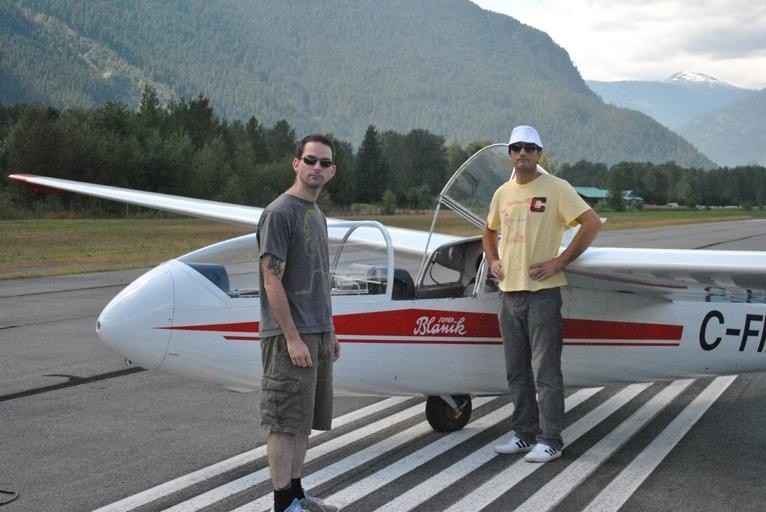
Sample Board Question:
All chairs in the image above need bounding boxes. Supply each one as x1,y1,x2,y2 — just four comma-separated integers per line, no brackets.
416,235,501,297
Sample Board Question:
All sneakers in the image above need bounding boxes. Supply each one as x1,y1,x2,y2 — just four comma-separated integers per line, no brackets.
524,442,562,462
297,488,340,512
495,437,537,455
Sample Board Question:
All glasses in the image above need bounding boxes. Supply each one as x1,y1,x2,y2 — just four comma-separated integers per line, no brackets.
511,142,541,153
300,155,333,167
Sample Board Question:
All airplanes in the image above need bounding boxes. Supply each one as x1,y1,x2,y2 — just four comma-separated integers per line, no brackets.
7,143,766,434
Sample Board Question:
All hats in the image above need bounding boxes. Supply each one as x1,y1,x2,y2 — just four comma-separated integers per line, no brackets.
509,126,543,149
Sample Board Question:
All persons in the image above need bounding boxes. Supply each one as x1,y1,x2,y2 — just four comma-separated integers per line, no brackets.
478,124,605,463
256,134,342,511
